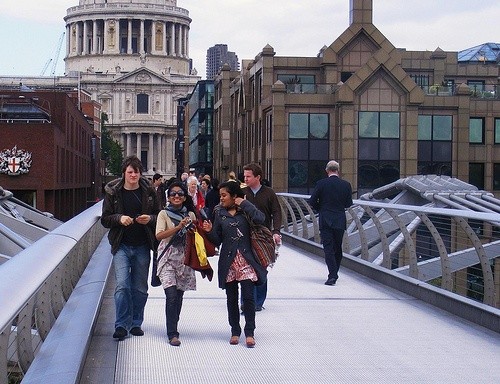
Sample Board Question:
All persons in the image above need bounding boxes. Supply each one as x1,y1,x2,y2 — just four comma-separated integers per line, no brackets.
155,182,199,346
239,163,283,316
308,160,354,285
202,181,265,348
147,168,271,256
100,155,162,339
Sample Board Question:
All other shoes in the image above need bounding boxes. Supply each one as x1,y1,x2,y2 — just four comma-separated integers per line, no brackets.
130,327,144,336
245,336,256,348
325,278,335,284
113,327,127,338
229,336,239,344
169,335,181,346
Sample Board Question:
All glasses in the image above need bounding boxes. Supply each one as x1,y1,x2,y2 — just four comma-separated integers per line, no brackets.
169,190,184,196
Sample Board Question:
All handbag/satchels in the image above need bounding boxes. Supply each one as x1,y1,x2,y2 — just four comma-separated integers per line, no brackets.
238,206,278,269
151,250,162,287
